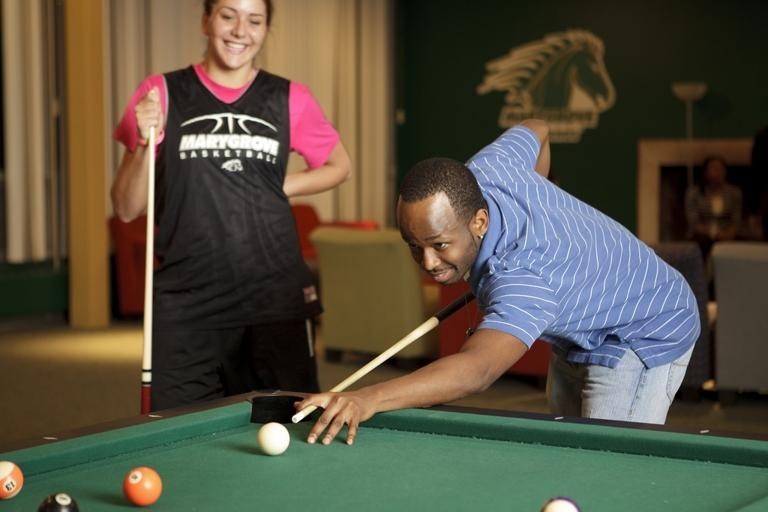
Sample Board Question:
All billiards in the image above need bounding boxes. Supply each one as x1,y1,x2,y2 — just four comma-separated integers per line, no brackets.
256,422,289,456
124,466,162,506
0,460,23,498
36,493,79,512
541,496,580,512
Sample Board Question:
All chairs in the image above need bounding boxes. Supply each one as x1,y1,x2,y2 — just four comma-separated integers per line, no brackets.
291,204,378,261
643,242,714,404
711,242,768,408
307,225,439,372
108,213,159,315
438,280,552,392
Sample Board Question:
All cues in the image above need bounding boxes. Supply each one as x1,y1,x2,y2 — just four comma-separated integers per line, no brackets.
292,290,477,424
141,89,157,414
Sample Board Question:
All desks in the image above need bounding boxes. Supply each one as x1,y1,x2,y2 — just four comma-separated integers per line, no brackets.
0,386,767,511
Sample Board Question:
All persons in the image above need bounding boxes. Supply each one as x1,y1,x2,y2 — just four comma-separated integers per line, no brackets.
111,0,354,412
683,156,744,247
294,119,702,447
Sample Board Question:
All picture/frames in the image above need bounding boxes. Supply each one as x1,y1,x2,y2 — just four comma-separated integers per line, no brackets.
636,138,754,246
